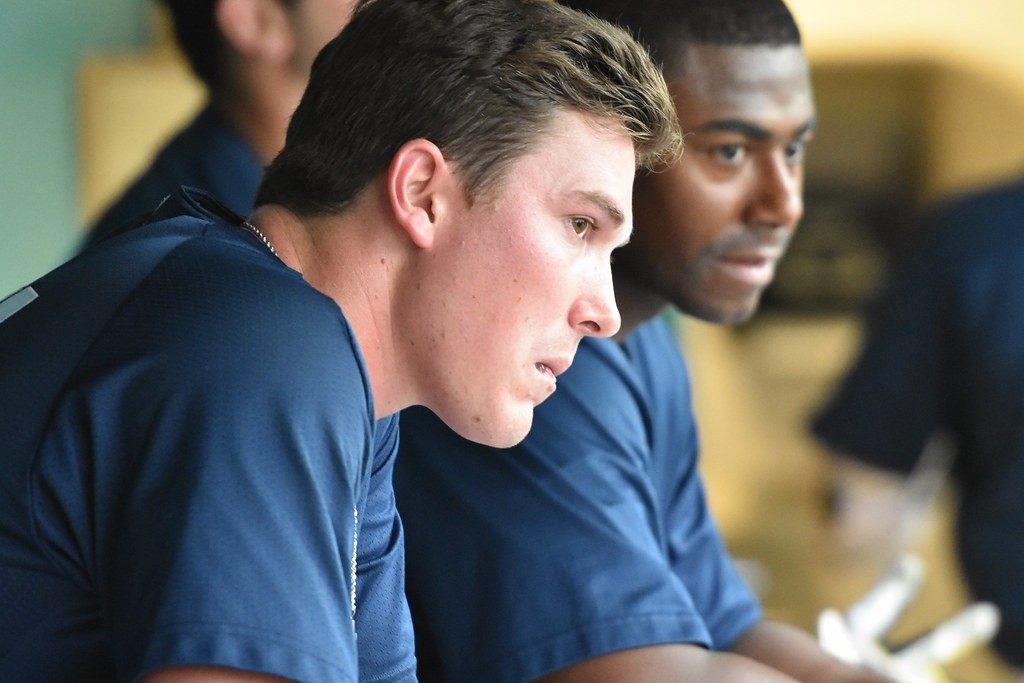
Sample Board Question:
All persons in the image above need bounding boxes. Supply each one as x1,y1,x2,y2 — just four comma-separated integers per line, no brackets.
75,0,364,264
0,0,683,682
803,172,1023,677
399,2,1009,683
